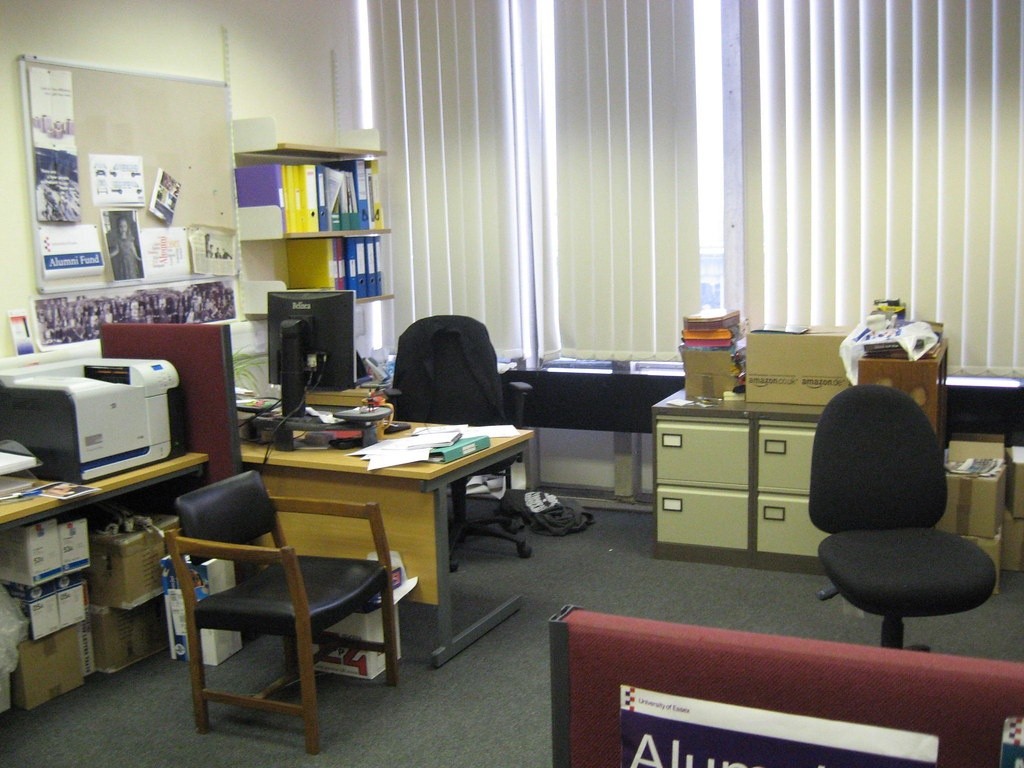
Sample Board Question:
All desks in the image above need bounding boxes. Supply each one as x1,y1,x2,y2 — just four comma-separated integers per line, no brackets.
240,419,534,667
0,452,209,533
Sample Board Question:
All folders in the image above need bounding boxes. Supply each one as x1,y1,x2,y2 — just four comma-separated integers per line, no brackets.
234,158,385,232
420,435,491,464
284,236,382,298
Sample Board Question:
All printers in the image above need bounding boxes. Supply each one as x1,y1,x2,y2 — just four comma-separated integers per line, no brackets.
0,353,188,485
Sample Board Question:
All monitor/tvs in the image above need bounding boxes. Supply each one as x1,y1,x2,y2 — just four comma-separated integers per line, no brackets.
266,290,356,421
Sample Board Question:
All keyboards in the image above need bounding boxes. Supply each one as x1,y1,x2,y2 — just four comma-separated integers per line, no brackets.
383,422,410,435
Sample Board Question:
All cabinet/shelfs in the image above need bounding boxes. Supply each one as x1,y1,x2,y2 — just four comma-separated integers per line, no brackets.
652,388,832,576
233,117,395,319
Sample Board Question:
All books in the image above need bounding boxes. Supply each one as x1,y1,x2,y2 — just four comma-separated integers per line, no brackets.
863,334,943,358
682,325,740,347
407,431,462,448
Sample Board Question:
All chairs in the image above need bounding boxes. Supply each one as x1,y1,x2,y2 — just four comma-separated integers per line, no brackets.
384,315,535,573
165,471,399,754
807,385,998,651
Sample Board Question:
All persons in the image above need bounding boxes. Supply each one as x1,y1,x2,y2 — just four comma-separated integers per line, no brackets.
109,216,142,280
37,279,234,345
207,245,232,259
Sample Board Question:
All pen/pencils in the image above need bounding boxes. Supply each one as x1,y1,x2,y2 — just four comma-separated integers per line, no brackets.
18,490,42,498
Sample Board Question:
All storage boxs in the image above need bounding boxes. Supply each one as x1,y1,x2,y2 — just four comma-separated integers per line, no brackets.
680,320,1024,594
1,511,243,713
312,551,419,680
